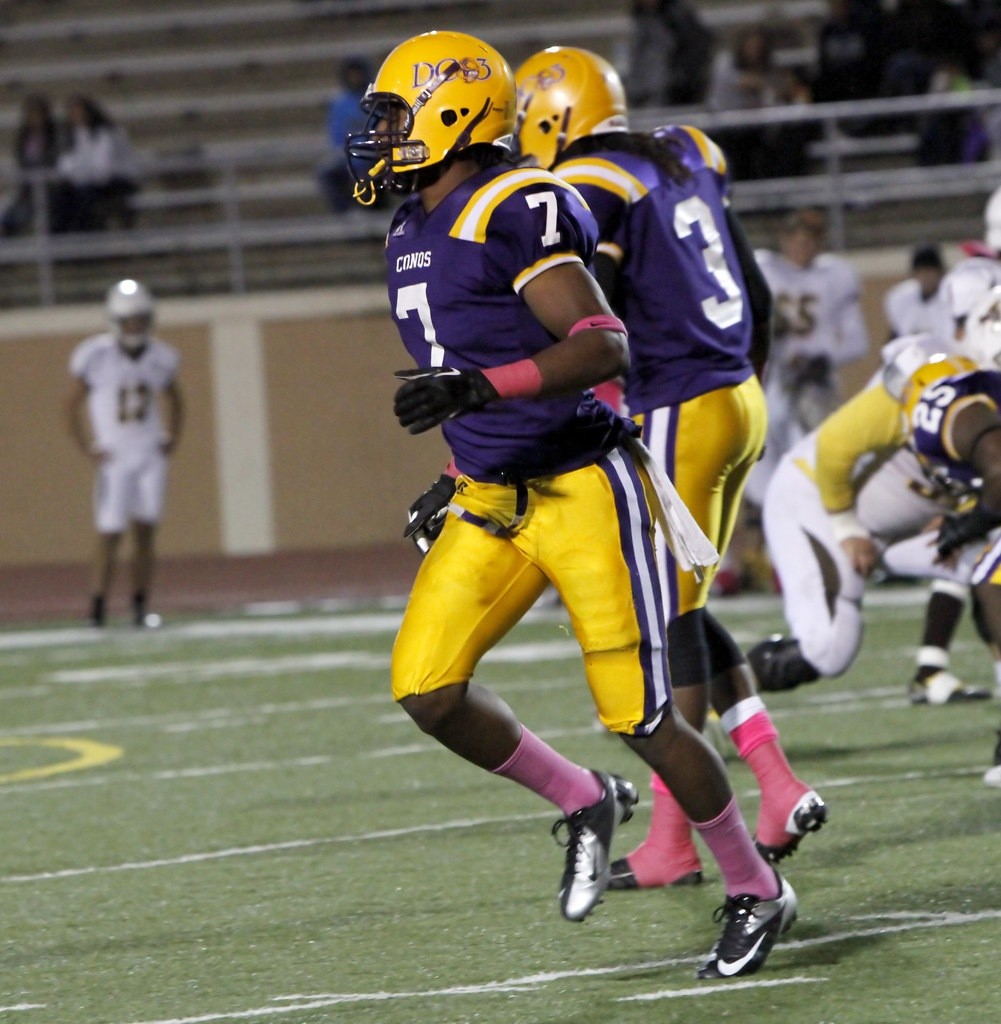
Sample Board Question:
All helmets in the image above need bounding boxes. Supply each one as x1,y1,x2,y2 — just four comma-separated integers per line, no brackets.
899,355,980,447
106,280,155,350
515,45,628,170
345,30,518,206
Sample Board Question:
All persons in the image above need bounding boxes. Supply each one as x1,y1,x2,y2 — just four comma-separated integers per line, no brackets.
59,277,186,624
505,46,825,890
0,95,208,238
346,31,799,981
542,0,1001,177
703,206,864,597
746,190,1001,790
314,56,391,209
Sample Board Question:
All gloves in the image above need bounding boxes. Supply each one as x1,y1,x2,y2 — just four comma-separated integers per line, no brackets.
404,473,456,557
393,366,500,434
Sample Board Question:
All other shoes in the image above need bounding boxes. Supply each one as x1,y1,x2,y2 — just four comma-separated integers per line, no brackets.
984,743,1001,787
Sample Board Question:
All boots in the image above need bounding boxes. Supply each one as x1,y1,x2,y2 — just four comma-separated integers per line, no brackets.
744,740,828,865
606,793,702,890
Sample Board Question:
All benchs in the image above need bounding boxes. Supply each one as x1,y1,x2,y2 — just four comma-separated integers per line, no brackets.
0,1,1001,295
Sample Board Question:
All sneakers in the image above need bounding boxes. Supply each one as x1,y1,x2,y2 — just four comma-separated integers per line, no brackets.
911,670,993,705
698,866,798,979
552,769,639,922
